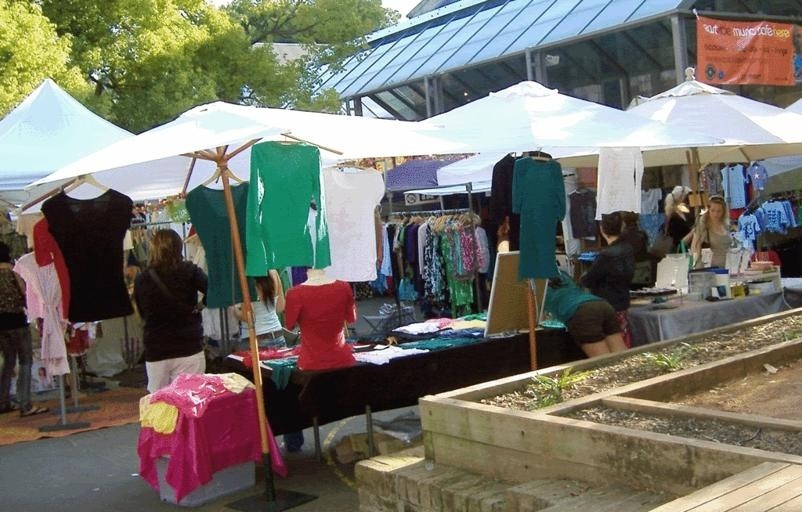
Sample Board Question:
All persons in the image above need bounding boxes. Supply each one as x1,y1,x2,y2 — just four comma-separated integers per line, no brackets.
0,243,48,418
579,213,639,350
535,266,630,360
230,269,287,347
690,195,732,271
134,229,209,393
284,267,357,370
665,185,696,253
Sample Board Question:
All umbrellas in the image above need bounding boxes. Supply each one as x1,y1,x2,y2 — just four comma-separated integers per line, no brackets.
419,78,742,371
625,70,802,270
25,100,445,502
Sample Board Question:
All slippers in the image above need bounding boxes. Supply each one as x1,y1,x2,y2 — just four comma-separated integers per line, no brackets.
0,401,49,416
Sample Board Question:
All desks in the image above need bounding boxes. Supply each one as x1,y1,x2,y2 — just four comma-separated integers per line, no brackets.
626,274,801,348
359,311,416,334
221,311,588,465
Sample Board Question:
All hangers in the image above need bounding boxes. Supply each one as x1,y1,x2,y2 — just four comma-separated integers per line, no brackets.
396,209,483,231
63,172,109,196
200,167,245,189
